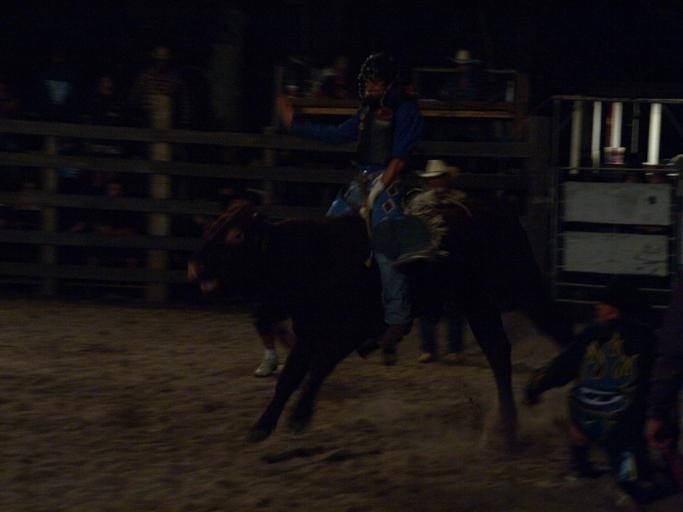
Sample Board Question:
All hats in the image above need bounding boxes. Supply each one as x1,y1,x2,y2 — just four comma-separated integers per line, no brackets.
413,157,460,182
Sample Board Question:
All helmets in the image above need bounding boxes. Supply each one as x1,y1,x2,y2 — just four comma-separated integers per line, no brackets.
354,51,403,109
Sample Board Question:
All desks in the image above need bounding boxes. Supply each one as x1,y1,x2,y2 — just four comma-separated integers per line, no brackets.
272,70,526,167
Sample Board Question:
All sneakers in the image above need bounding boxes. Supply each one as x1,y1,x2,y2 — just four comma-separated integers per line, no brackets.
416,351,434,365
446,353,465,365
250,355,281,380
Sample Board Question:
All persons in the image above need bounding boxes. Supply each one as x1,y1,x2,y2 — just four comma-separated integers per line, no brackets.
516,279,661,511
403,158,473,368
1,1,682,307
275,51,443,272
641,273,682,457
355,319,413,368
220,182,303,380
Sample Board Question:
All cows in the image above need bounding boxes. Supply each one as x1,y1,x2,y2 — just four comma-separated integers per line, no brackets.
243,200,557,443
